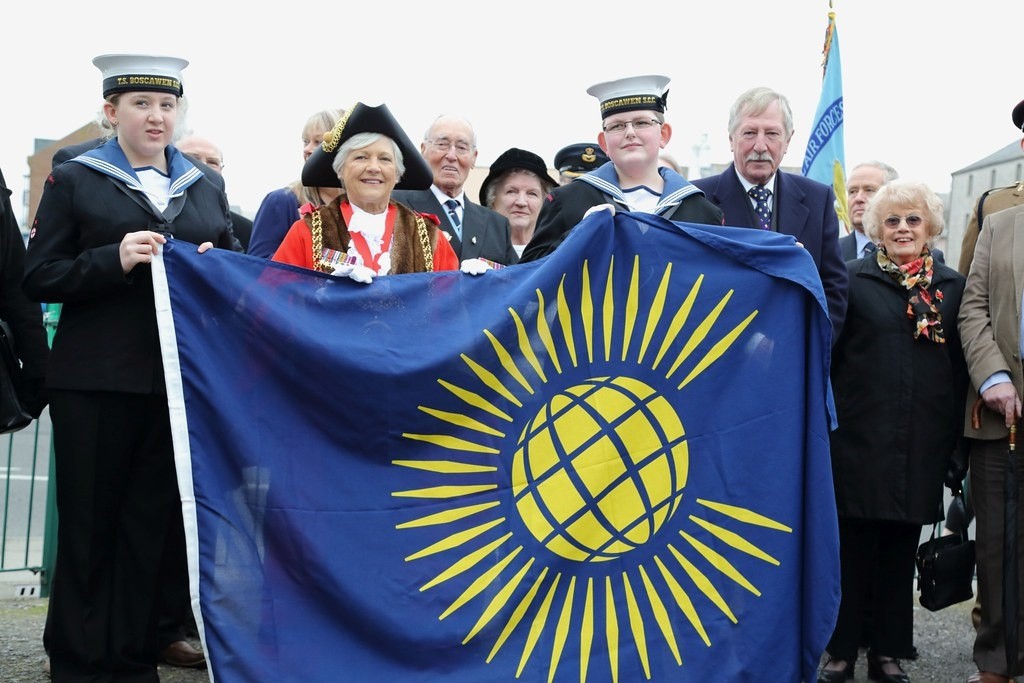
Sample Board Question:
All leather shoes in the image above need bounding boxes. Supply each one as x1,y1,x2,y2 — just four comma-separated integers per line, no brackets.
966,669,1009,683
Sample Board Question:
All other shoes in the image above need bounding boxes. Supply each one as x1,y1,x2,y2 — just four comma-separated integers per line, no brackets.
167,638,206,666
818,653,860,682
866,654,909,682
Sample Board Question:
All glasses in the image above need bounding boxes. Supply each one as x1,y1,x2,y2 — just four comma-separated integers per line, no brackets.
880,215,926,229
427,140,472,153
206,159,225,170
603,117,662,133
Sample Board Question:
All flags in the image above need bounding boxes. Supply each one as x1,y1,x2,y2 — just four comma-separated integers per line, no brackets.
152,212,842,683
803,18,850,238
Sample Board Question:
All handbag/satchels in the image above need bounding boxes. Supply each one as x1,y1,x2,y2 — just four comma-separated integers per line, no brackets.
0,319,46,435
918,480,974,612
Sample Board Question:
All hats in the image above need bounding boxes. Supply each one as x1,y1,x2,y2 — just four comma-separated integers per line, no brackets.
92,52,189,100
553,143,612,179
301,102,435,192
479,149,559,207
585,73,671,119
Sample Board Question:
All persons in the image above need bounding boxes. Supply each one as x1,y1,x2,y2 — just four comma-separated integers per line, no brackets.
841,161,899,260
391,115,519,274
24,54,242,683
249,102,461,350
688,88,848,350
553,143,612,185
244,109,351,259
175,137,252,251
1,174,51,432
818,181,967,682
958,98,1023,683
517,77,724,264
51,95,188,170
479,148,561,259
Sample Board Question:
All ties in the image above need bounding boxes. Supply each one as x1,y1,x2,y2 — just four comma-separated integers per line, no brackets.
445,199,462,233
747,185,774,231
863,242,878,258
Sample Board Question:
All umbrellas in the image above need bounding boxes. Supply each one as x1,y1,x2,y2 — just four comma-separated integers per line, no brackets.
972,399,1018,683
